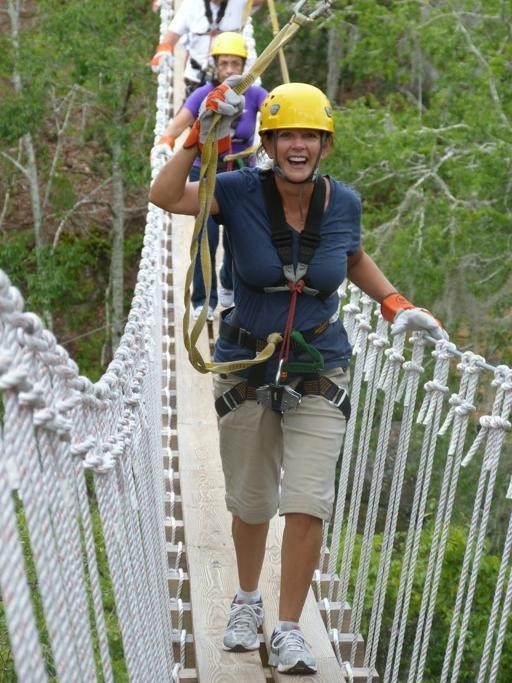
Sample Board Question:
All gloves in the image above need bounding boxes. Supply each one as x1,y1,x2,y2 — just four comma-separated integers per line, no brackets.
185,75,245,150
150,135,175,159
383,294,451,341
153,0,171,14
151,43,173,73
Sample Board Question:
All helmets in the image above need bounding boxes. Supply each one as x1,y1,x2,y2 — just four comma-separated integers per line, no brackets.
212,30,247,60
258,83,335,135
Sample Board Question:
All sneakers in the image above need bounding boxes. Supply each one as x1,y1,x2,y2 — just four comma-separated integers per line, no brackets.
193,305,216,323
269,627,318,673
219,283,235,307
222,593,265,652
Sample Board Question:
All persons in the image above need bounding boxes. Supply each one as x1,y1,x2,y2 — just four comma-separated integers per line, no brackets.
149,30,274,322
147,0,260,102
149,77,448,674
152,0,259,62
